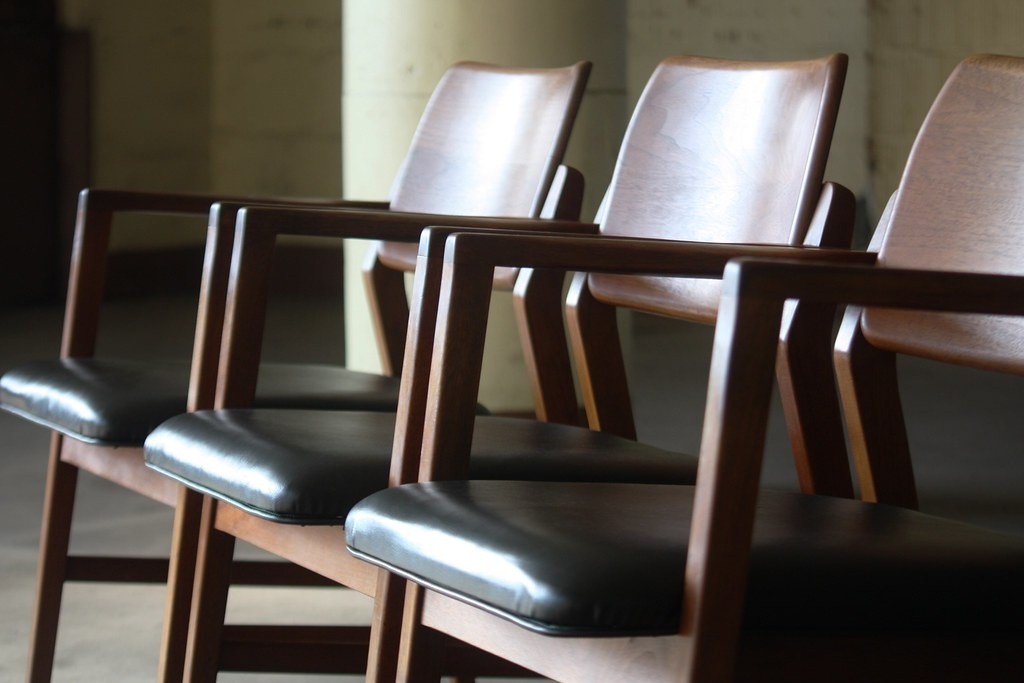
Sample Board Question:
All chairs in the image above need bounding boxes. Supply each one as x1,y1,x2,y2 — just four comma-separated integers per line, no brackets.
343,48,1024,683
140,53,857,683
0,60,583,683
